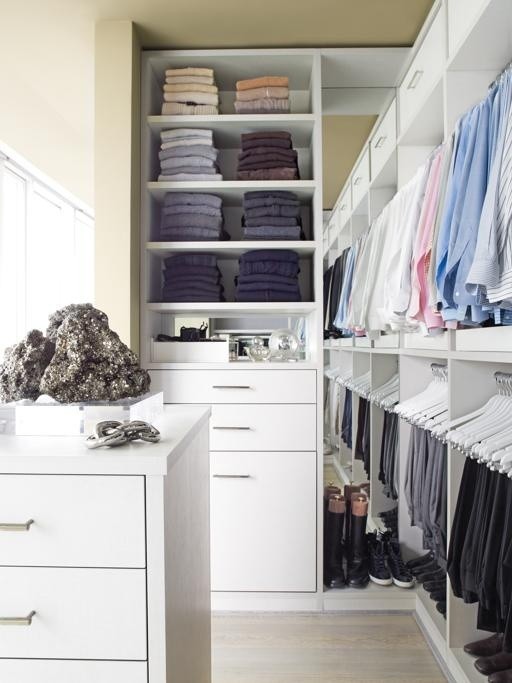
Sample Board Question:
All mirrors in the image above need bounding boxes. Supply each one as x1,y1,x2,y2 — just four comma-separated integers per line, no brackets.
324,48,405,614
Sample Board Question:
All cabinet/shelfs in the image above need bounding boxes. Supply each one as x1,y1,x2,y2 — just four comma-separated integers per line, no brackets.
140,49,320,366
1,403,212,683
141,364,323,613
406,1,511,683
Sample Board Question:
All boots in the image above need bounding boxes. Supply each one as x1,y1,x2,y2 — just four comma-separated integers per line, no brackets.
323,492,415,588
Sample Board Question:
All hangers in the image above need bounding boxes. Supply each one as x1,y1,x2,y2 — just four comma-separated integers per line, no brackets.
324,362,399,416
395,363,511,481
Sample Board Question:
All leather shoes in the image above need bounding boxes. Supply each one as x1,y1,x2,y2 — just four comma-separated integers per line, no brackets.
463,631,512,683
407,550,446,618
